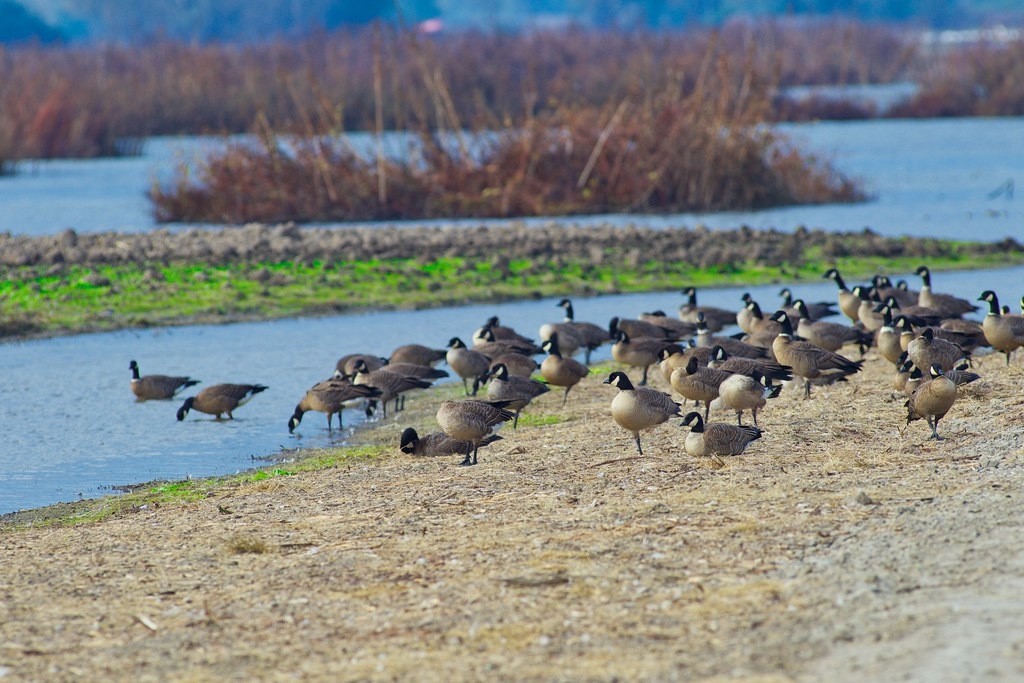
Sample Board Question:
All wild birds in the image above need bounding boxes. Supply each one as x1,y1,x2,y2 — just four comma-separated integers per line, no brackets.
176,382,269,422
128,360,191,400
288,265,1023,468
